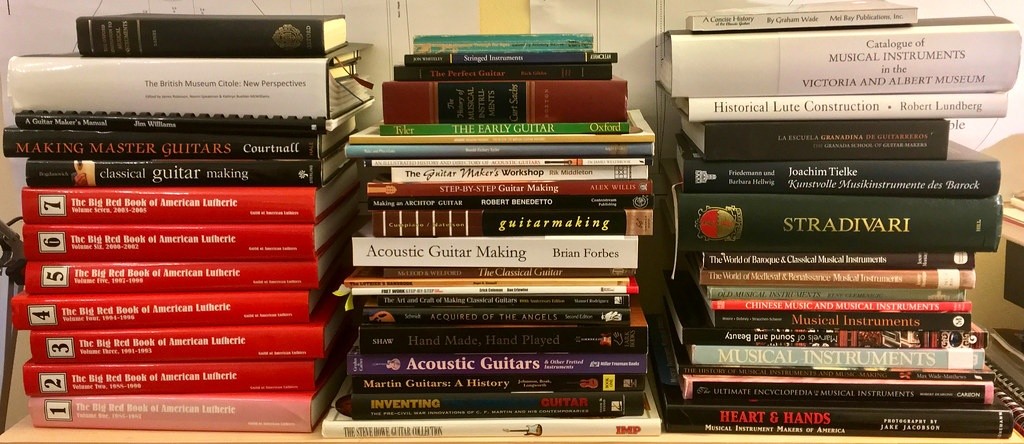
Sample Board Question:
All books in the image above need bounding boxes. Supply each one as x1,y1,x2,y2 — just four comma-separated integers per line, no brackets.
319,33,665,438
4,14,377,434
656,0,1024,439
